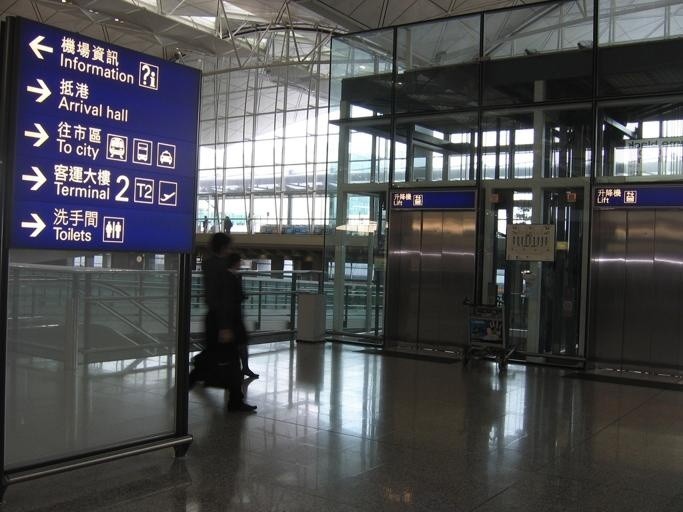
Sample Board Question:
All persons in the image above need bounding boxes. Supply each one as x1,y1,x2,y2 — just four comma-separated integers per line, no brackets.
203,216,208,234
161,231,259,413
224,216,233,233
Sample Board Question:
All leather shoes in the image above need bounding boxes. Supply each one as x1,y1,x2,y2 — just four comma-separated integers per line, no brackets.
242,367,259,377
228,398,257,412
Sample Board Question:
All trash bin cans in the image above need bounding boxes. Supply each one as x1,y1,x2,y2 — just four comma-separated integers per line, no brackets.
294,290,328,345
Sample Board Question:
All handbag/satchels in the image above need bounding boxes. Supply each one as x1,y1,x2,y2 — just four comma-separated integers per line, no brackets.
202,361,235,388
